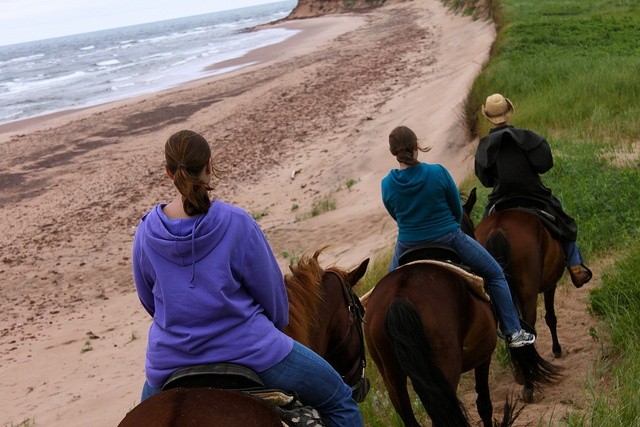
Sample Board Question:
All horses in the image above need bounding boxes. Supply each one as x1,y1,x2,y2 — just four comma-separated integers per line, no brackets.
473,206,567,403
363,186,497,426
116,242,370,427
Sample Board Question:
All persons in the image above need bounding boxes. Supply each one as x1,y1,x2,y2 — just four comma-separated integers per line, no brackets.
380,126,535,350
130,130,362,423
473,92,594,288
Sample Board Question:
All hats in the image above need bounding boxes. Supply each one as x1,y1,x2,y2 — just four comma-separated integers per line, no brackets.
481,94,515,125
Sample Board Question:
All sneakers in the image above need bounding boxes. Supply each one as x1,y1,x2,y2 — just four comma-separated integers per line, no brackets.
506,328,536,348
567,262,591,285
